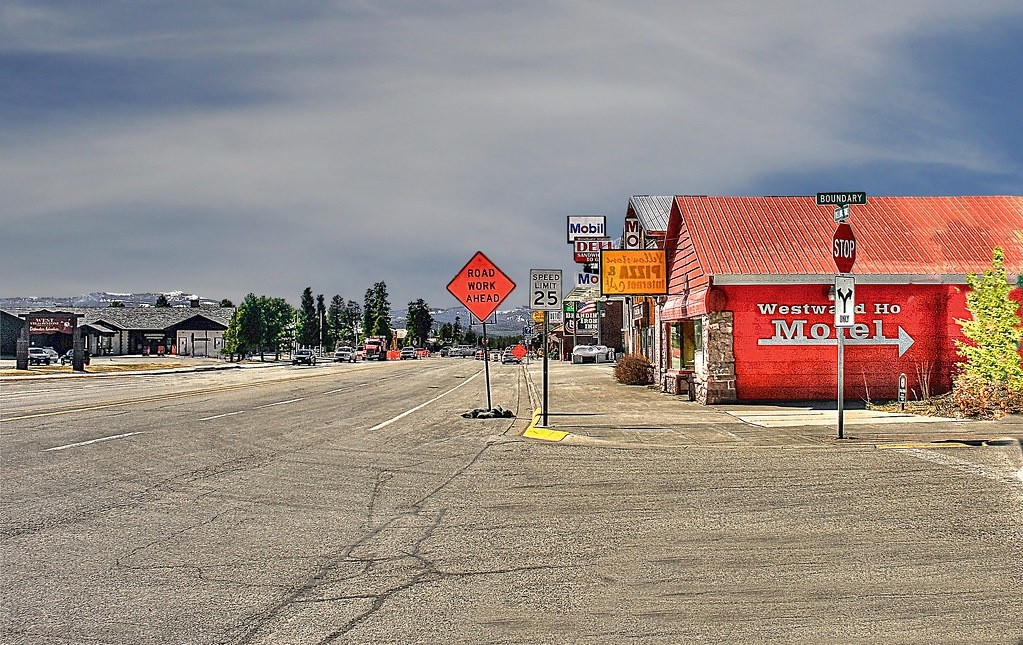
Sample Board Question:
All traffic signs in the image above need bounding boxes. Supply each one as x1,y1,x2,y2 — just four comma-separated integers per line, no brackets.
833,276,856,329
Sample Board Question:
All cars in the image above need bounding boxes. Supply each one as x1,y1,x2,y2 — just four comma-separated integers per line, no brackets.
440,344,520,365
44,348,59,363
291,349,317,366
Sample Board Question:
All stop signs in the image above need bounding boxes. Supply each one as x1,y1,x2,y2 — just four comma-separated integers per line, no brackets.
831,223,857,274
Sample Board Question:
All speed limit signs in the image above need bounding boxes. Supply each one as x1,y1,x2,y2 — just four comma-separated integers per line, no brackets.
527,268,564,312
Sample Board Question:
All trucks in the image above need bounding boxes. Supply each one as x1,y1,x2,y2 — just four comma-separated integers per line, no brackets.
362,337,387,361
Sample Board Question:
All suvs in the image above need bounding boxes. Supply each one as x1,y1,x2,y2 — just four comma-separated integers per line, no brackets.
60,349,91,365
333,347,357,362
399,346,417,360
28,347,51,366
